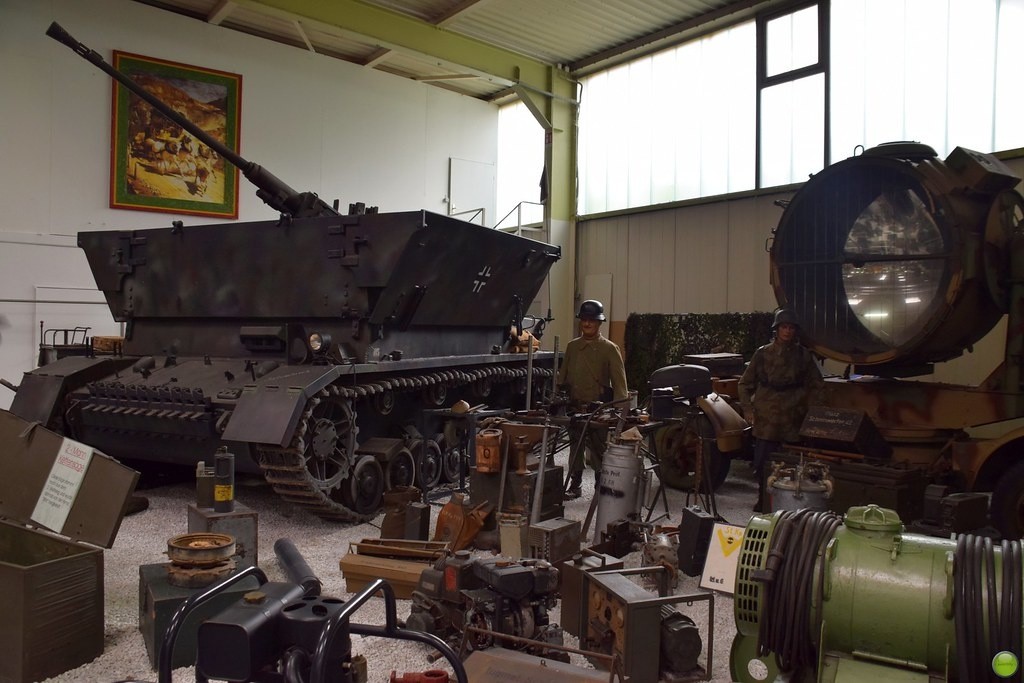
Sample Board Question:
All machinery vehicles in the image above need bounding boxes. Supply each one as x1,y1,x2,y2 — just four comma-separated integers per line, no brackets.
7,19,570,527
644,140,1024,539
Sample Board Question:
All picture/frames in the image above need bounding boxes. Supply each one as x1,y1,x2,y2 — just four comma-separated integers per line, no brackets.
110,50,243,220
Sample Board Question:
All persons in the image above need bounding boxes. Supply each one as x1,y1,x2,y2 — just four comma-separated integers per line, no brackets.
738,310,825,514
555,300,628,501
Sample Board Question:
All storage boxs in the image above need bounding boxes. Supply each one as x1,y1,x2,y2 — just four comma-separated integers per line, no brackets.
0,408,141,683
